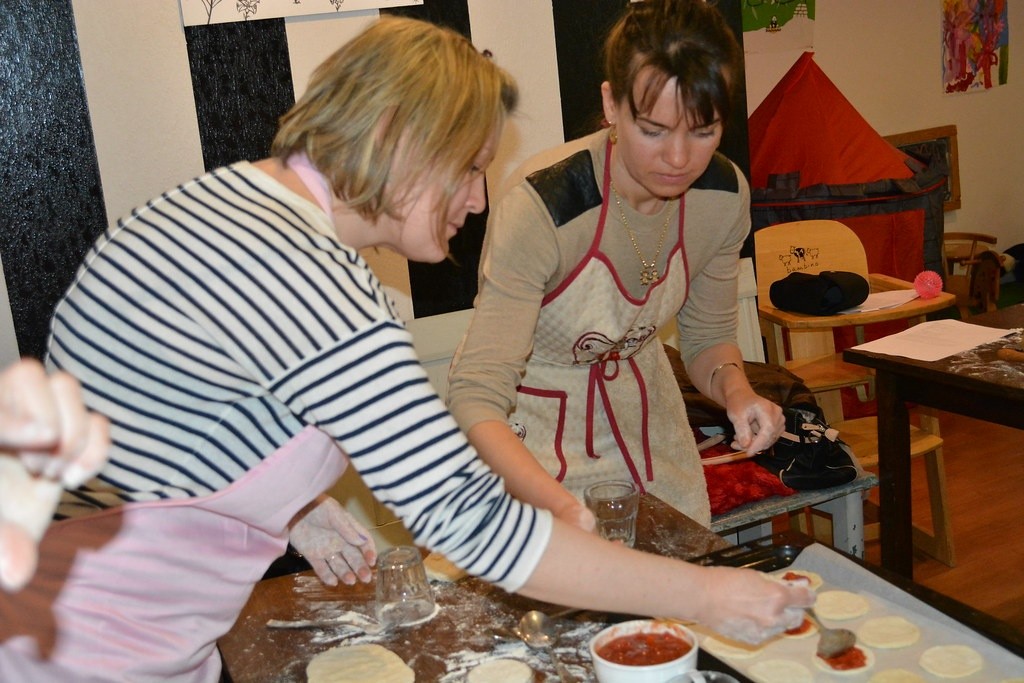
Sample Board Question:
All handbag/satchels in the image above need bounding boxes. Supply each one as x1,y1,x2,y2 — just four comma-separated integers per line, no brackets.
696,401,857,491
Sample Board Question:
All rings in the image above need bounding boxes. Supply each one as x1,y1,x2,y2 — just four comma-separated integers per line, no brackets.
328,555,338,562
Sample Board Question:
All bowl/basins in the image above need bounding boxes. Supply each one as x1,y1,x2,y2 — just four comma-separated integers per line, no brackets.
665,671,741,683
590,619,698,683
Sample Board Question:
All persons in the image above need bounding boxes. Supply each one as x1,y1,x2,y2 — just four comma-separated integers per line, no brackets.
0,14,815,683
446,0,787,535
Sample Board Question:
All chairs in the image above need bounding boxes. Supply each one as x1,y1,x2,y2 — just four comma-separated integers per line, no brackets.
942,232,1006,319
754,220,960,566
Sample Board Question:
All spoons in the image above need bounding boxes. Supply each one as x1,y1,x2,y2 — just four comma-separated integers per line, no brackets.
517,610,578,683
788,583,857,658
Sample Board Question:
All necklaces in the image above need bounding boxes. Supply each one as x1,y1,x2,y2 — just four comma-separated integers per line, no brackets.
611,178,675,286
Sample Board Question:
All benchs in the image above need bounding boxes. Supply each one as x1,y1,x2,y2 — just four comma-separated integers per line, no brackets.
325,258,880,561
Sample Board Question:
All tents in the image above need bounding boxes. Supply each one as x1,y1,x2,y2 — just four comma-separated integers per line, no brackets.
748,53,947,419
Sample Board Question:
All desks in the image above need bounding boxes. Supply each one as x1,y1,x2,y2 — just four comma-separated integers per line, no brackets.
842,304,1024,579
215,489,759,683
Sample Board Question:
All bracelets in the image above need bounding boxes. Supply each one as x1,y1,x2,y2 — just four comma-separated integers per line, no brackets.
711,362,741,392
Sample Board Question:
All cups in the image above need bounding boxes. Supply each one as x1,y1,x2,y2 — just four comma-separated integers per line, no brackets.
584,480,639,549
376,545,436,625
